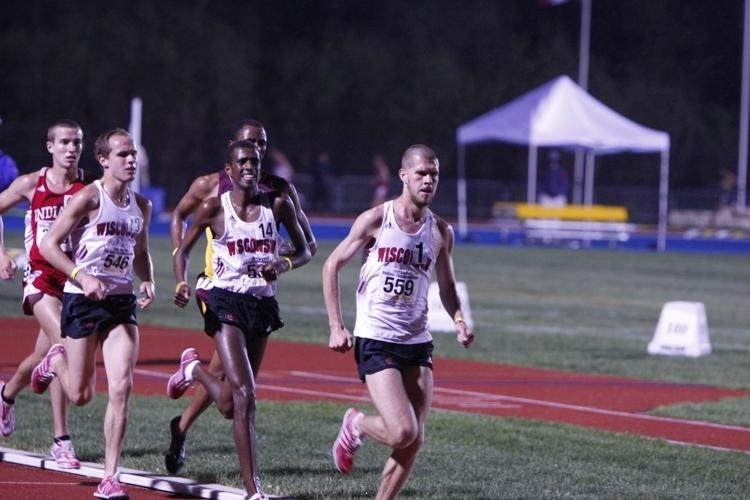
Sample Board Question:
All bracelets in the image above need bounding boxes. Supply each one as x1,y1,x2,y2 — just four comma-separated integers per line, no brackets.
70,267,81,280
172,247,178,256
280,256,292,272
454,316,464,322
175,280,187,293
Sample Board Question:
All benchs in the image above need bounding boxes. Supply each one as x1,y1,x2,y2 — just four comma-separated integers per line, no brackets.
466,199,640,253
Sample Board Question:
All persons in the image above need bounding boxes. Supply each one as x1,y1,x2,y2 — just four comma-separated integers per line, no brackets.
267,146,293,182
369,154,390,209
321,142,476,500
716,166,737,209
0,116,95,471
30,125,157,500
538,151,570,207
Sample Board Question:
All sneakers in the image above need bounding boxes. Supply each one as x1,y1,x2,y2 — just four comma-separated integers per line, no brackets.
332,407,364,474
93,473,127,499
167,348,201,398
31,345,65,394
165,416,186,473
0,381,15,436
50,439,80,468
